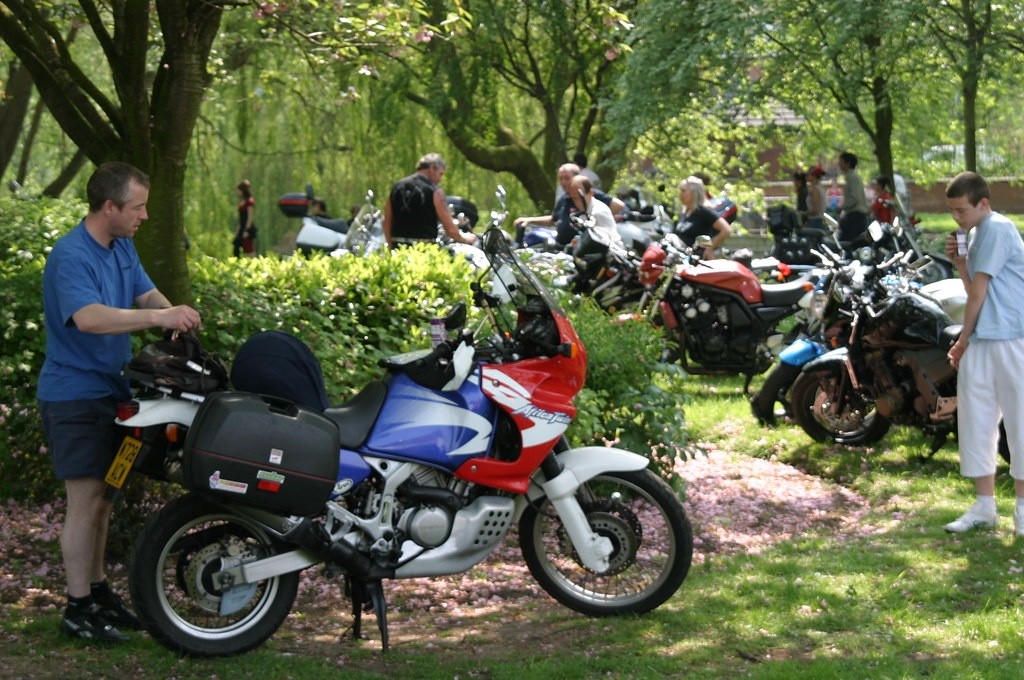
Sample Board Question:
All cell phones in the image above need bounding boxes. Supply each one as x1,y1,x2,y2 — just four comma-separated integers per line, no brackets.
955,230,967,258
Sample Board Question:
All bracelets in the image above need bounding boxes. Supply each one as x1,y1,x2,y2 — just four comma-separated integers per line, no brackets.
244,227,251,232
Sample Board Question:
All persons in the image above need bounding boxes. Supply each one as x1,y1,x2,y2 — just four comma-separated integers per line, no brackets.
871,164,906,227
35,160,200,647
383,153,476,252
311,197,361,227
792,161,827,227
945,171,1024,535
838,153,868,213
513,153,627,259
232,179,255,259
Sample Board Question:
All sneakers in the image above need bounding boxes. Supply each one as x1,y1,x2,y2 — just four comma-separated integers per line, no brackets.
942,503,999,533
94,590,143,631
1012,504,1024,537
61,604,130,647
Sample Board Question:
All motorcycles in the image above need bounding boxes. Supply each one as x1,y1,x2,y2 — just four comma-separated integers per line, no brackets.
276,181,1013,466
103,227,694,659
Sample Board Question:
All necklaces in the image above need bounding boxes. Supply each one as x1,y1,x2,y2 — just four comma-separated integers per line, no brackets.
675,172,731,260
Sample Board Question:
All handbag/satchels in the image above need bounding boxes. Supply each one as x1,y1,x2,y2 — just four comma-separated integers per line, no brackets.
129,326,228,391
